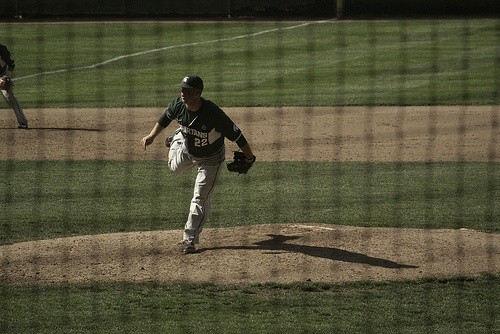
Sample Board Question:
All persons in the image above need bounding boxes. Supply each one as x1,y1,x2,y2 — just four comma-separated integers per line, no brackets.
140,76,255,254
0,44,28,129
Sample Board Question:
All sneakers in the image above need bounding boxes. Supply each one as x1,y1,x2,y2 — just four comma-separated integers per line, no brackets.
165,136,173,147
182,241,196,254
18,124,28,129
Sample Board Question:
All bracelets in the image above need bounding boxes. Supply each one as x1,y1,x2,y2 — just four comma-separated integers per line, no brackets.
246,155,254,160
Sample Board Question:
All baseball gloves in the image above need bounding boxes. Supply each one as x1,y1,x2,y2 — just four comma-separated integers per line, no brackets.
226,149,257,176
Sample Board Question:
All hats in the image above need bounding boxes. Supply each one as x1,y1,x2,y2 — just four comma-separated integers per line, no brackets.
175,75,203,90
